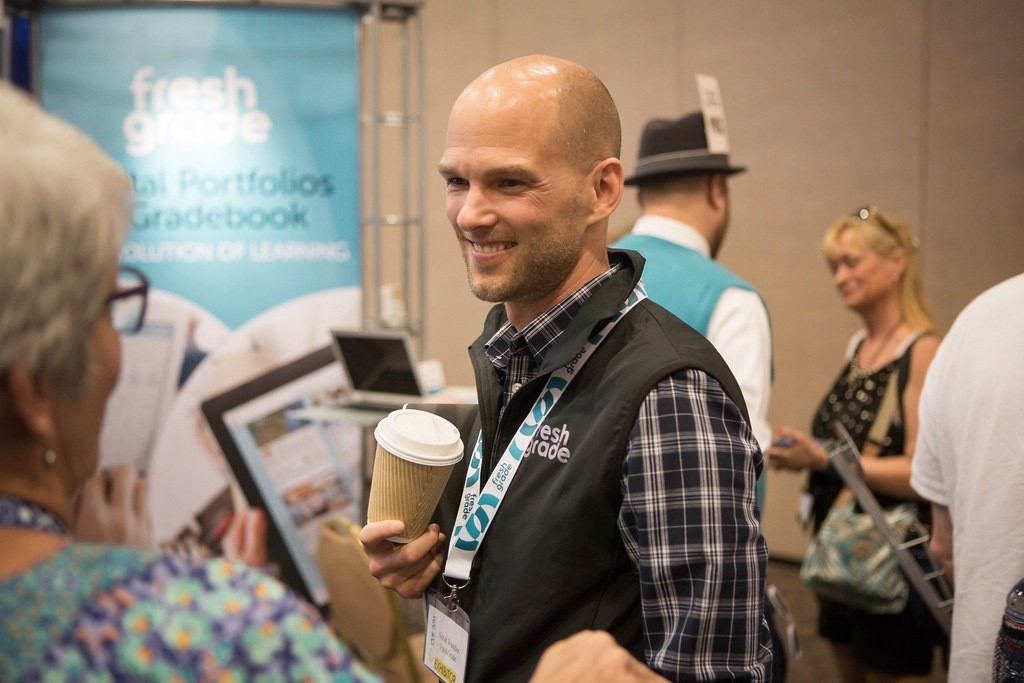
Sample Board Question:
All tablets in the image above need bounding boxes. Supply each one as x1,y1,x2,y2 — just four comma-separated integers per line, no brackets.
198,347,367,621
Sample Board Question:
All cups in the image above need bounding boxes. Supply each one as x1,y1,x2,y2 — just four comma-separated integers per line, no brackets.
366,409,464,542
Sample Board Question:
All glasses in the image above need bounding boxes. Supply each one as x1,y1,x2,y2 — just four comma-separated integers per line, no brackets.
854,206,902,245
107,267,149,333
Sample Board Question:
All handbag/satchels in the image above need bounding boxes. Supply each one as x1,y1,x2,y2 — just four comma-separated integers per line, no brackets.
800,372,918,612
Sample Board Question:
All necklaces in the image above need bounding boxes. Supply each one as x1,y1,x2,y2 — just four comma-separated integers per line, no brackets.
861,316,905,371
4,489,71,535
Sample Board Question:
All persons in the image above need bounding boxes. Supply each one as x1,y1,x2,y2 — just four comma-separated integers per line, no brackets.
768,206,939,682
0,76,376,683
909,271,1024,683
358,53,772,681
606,108,771,512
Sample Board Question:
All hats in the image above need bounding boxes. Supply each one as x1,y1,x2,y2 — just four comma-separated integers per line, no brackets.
623,111,746,186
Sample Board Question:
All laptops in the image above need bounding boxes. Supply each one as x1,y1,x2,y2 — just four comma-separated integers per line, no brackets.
329,329,464,407
